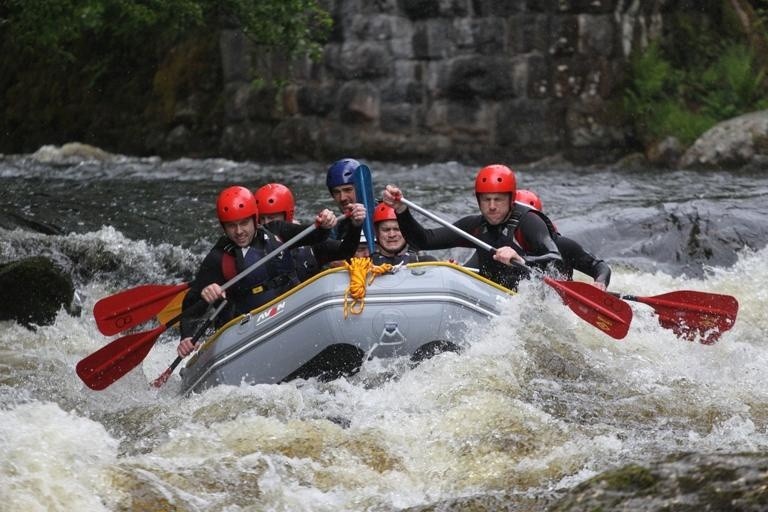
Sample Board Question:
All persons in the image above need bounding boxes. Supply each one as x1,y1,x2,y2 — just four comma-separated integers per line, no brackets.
384,165,565,291
178,158,370,358
373,203,438,267
516,190,611,292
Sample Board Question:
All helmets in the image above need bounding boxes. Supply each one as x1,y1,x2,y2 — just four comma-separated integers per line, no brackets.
373,201,406,224
253,183,295,225
326,158,362,192
508,189,542,214
474,164,517,203
216,185,259,224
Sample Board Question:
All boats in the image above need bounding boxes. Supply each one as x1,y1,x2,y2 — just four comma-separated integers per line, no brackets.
180,260,518,402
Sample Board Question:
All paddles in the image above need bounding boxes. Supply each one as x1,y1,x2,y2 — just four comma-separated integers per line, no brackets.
606,290,738,332
622,299,722,345
93,279,197,336
354,163,377,266
76,214,323,391
393,192,633,340
156,282,191,324
